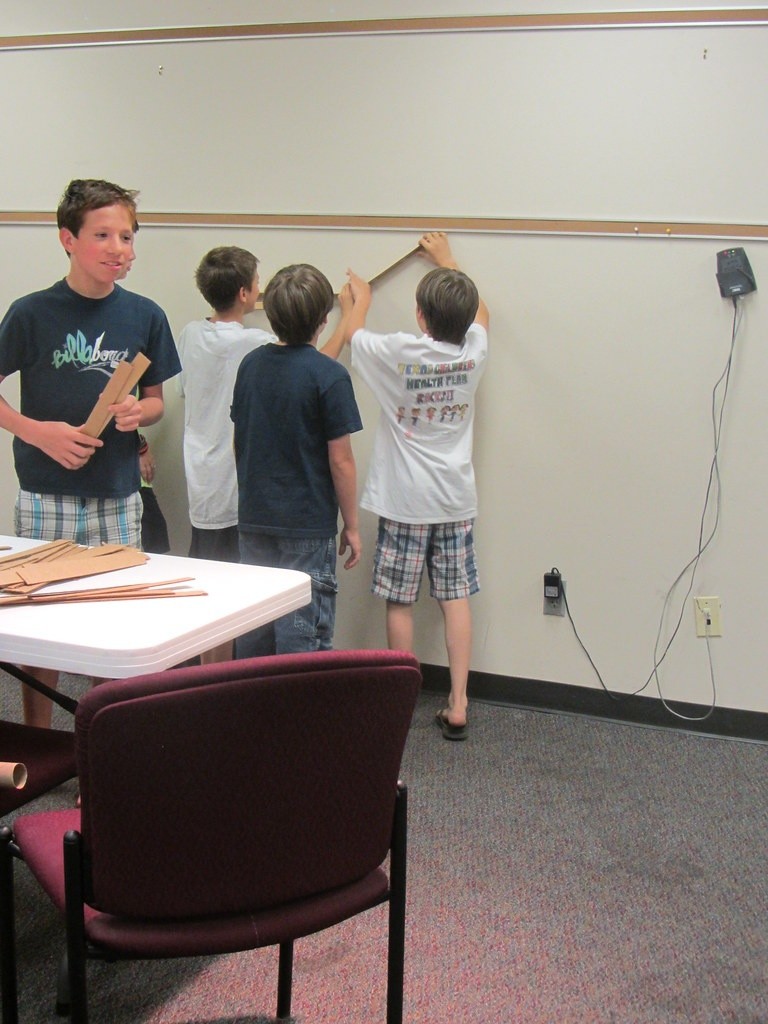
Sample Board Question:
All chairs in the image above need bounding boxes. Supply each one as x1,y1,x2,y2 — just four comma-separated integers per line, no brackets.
0,647,423,1024
0,719,79,818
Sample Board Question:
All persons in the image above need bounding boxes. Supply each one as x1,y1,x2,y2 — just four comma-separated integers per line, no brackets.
176,246,354,670
0,178,183,729
346,232,489,741
230,264,364,662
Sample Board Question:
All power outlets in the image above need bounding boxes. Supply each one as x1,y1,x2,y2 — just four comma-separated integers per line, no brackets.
543,580,568,617
693,596,724,638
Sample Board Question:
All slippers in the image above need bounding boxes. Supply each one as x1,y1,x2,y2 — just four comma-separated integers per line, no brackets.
436,709,470,741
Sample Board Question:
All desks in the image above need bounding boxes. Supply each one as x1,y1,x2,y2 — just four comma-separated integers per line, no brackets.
0,534,312,715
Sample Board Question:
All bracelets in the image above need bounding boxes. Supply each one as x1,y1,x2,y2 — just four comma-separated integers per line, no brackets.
139,435,148,454
